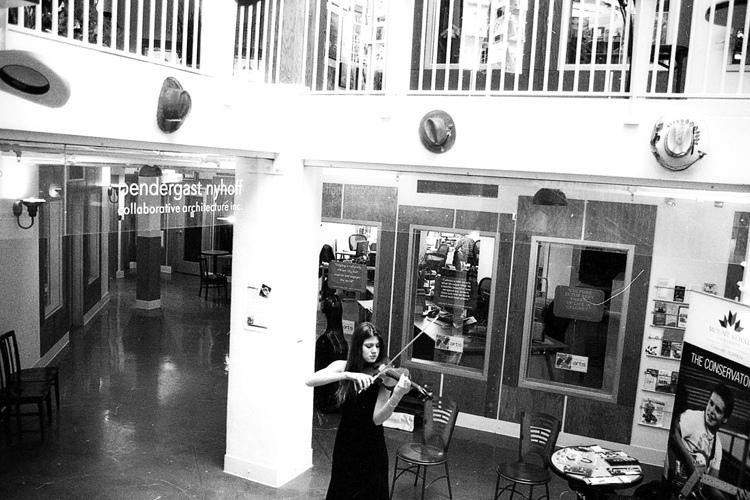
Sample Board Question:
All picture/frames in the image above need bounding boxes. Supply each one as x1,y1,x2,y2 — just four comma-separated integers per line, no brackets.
726,27,750,73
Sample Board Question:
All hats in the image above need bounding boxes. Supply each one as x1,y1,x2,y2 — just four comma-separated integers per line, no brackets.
157,76,191,133
650,111,707,171
419,110,456,154
0,49,71,108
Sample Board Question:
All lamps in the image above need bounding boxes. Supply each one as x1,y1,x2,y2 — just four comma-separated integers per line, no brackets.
13,197,47,230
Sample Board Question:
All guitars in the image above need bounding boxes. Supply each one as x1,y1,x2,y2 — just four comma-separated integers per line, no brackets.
666,438,750,499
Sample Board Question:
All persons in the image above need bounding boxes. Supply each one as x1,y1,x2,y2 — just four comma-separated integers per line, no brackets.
306,321,414,500
669,383,750,500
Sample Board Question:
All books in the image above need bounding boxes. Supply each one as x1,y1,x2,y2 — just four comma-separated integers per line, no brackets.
558,444,643,486
640,286,689,426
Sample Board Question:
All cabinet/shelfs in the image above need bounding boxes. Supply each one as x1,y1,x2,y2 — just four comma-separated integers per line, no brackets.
636,285,689,431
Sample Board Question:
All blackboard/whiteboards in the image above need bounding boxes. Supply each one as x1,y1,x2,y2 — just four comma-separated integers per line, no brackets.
328,262,366,293
433,276,477,310
554,285,605,322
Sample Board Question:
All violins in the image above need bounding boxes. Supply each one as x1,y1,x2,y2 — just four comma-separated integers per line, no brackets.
370,358,434,403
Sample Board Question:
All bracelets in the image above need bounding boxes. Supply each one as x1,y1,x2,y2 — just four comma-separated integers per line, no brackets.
387,398,399,408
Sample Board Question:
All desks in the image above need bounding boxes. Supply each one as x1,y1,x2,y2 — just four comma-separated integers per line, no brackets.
199,249,230,277
357,286,569,383
549,444,645,500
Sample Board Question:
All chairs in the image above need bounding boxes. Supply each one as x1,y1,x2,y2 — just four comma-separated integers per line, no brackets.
390,397,461,500
437,244,448,261
1,329,61,441
320,234,376,298
198,257,227,301
495,409,561,500
617,0,688,99
